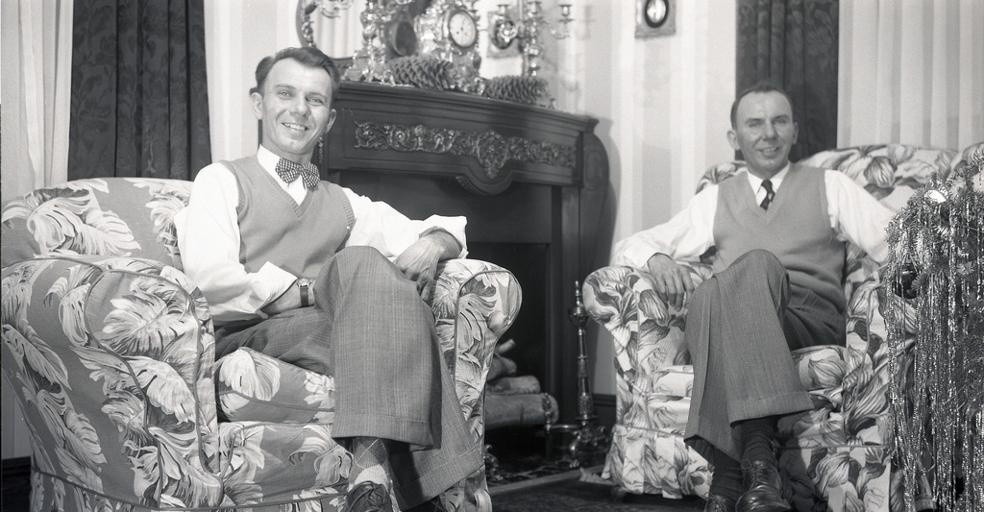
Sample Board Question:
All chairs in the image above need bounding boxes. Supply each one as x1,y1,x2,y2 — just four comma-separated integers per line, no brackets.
582,145,963,512
3,177,525,511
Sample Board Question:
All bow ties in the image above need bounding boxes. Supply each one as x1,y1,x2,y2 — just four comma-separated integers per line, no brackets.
275,156,321,188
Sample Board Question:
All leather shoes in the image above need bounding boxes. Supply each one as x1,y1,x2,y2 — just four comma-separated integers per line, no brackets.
339,480,393,512
703,458,794,512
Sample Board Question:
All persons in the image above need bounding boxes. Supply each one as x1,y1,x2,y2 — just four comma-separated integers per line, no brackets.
173,45,493,512
609,80,901,512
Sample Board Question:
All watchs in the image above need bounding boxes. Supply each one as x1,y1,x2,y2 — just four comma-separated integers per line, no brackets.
294,277,315,307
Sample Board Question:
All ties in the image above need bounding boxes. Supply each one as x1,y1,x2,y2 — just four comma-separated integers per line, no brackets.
759,179,775,210
